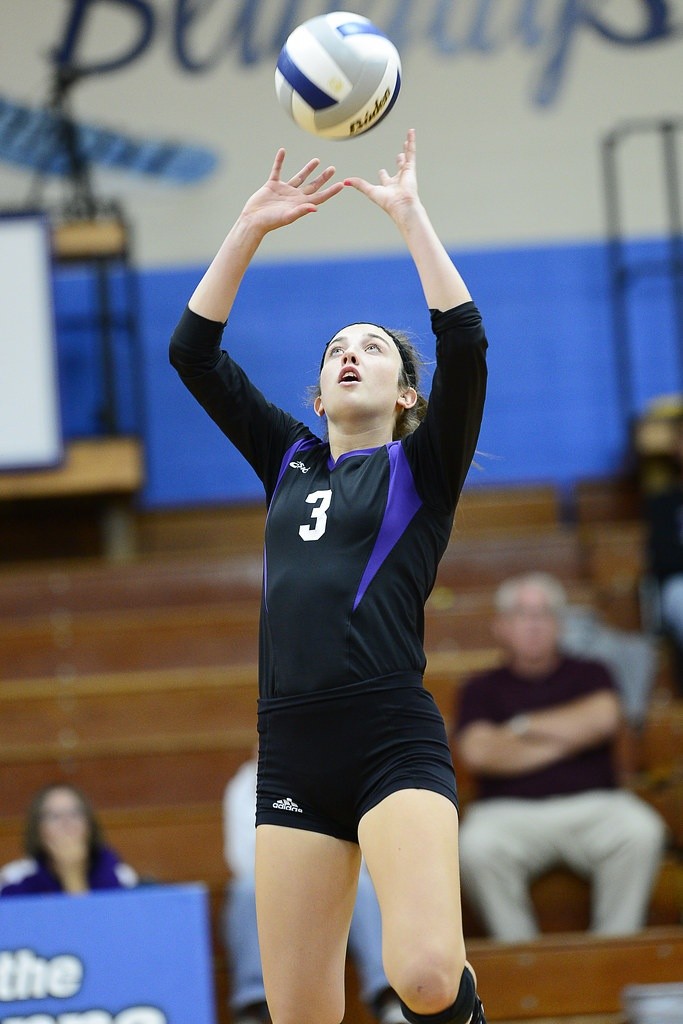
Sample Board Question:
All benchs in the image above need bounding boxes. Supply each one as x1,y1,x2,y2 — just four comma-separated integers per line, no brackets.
0,474,683,1024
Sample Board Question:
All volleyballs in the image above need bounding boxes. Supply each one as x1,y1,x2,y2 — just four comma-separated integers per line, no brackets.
273,9,404,143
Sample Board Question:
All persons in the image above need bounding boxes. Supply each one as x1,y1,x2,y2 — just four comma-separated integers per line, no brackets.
170,128,489,1024
223,751,412,1024
633,399,683,644
0,784,141,897
453,573,666,944
